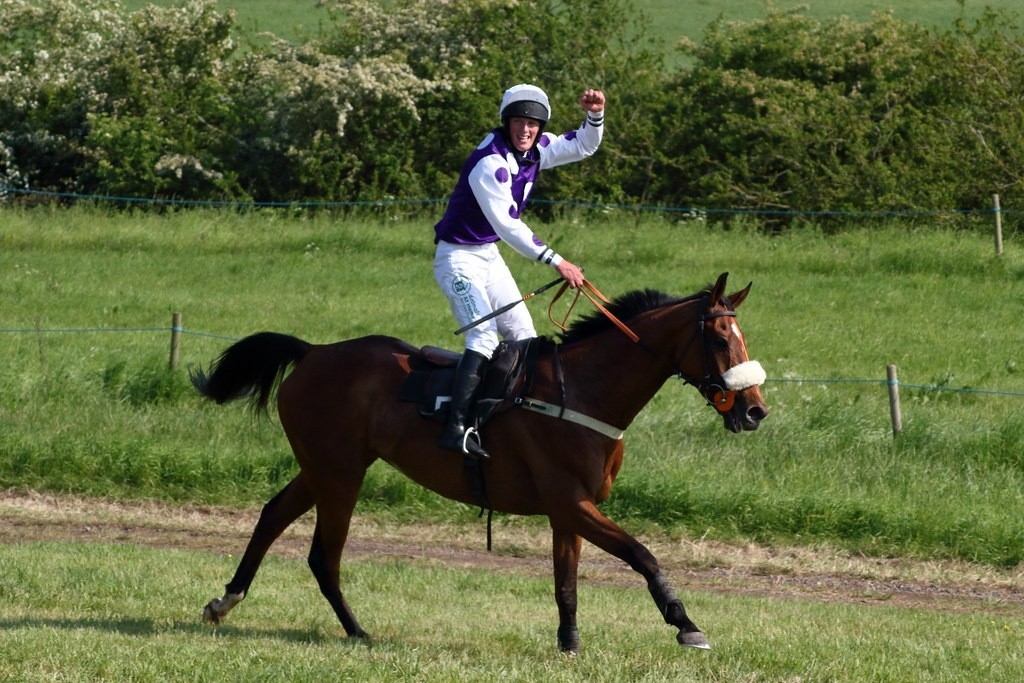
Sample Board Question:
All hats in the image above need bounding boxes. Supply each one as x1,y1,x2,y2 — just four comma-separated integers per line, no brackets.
500,84,551,121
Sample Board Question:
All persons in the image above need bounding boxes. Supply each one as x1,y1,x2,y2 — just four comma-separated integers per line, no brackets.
434,84,605,460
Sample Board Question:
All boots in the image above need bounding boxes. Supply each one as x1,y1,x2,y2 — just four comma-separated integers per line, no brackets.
437,348,490,459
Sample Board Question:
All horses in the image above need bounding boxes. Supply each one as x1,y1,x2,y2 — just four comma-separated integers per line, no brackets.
186,271,771,656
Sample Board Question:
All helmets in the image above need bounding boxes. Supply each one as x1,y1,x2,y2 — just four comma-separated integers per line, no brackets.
501,101,548,145
